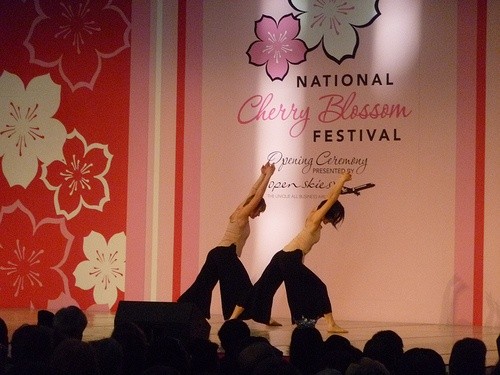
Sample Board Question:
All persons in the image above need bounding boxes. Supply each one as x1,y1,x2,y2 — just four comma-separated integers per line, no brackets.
177,161,283,326
0,305,499,374
229,171,349,334
